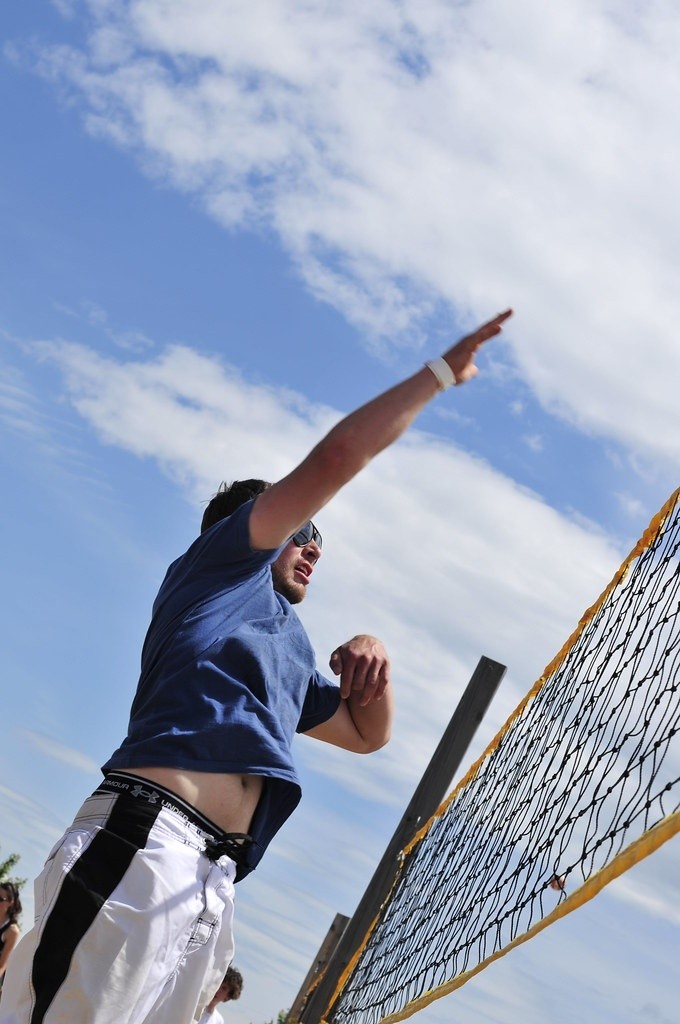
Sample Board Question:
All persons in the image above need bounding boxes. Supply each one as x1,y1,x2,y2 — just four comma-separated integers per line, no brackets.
0,307,516,1024
198,967,243,1024
0,881,23,994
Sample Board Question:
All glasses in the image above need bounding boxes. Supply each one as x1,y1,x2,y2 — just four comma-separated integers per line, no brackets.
0,897,8,902
293,519,323,566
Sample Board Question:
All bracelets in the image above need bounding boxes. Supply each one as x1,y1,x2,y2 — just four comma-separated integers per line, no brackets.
425,356,456,390
